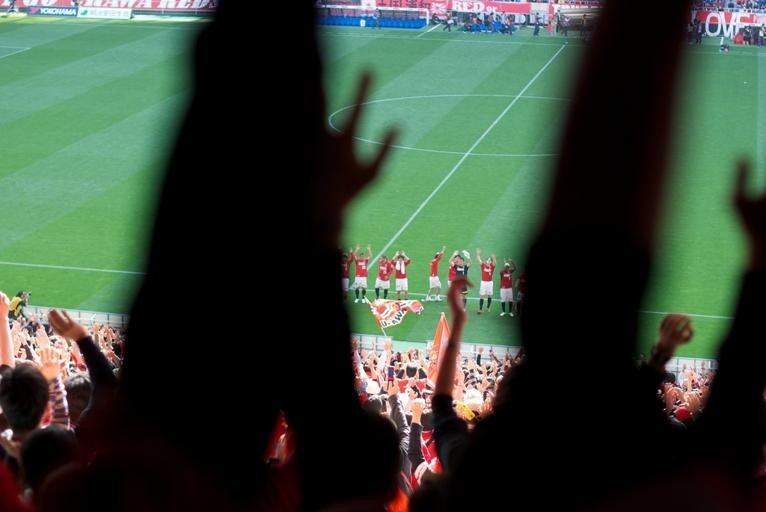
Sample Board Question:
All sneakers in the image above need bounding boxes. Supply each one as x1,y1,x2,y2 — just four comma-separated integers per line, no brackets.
344,295,516,319
